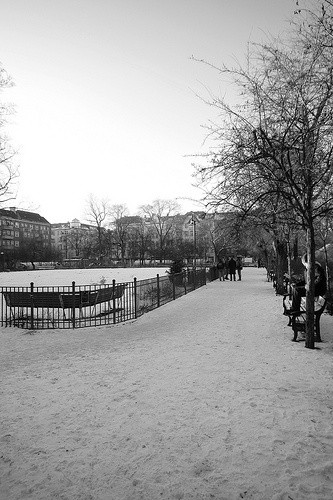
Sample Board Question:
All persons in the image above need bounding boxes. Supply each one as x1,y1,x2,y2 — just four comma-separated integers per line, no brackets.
284,252,327,317
236,257,243,281
224,257,230,280
217,258,225,281
228,257,237,281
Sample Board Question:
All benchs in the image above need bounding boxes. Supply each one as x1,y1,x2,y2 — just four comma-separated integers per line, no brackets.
282,291,327,342
